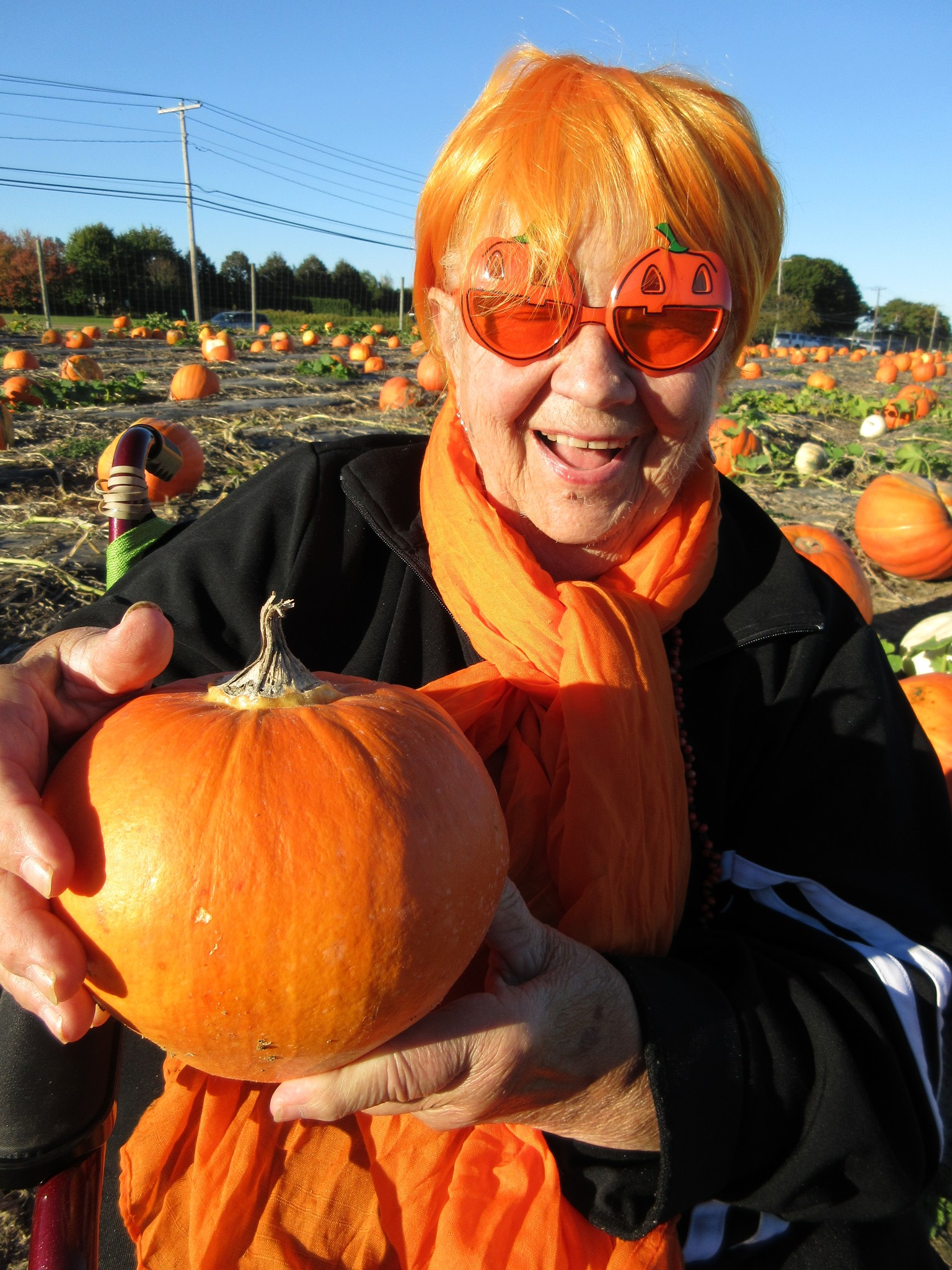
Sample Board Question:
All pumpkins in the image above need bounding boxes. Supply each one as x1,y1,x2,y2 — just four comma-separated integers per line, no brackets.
40,594,508,1094
853,466,952,582
83,416,203,511
891,671,951,794
779,522,873,632
0,263,952,439
692,410,757,477
894,608,952,683
932,475,952,518
792,443,829,479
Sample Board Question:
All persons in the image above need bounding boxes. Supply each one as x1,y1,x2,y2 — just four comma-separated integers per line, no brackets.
0,43,952,1270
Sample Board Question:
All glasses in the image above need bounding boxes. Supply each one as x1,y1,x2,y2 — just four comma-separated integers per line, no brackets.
432,219,751,378
